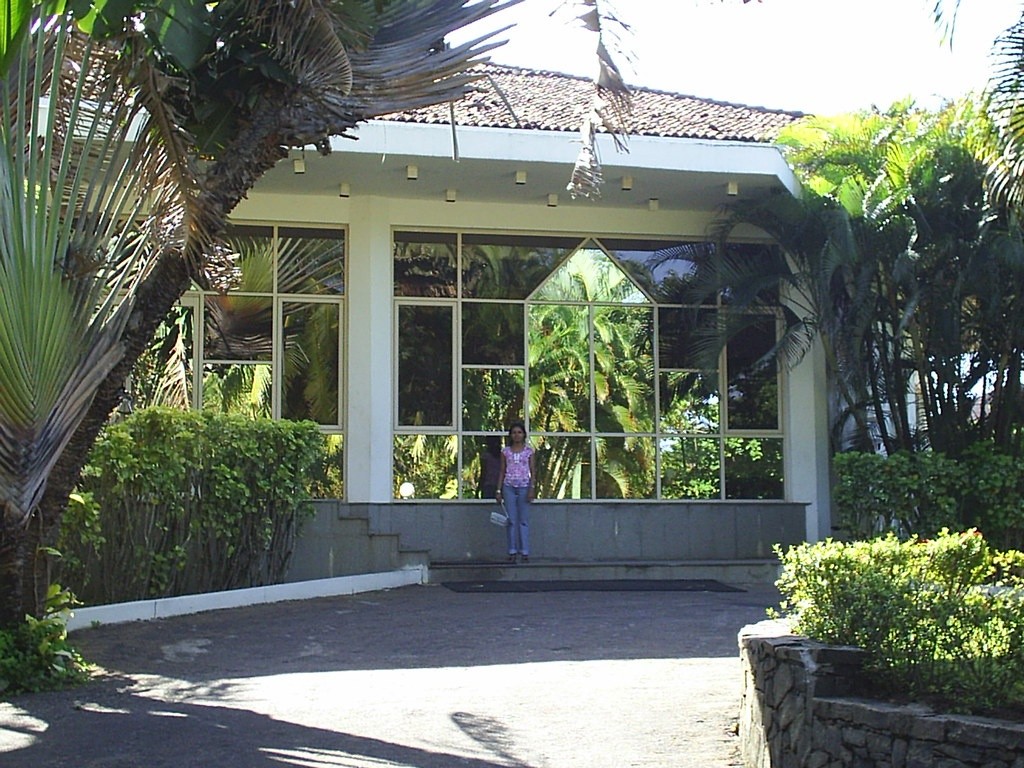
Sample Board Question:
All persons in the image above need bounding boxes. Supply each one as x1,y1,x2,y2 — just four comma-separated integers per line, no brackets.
494,425,536,564
475,435,503,498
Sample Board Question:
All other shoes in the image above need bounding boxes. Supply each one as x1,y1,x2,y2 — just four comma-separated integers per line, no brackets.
506,554,516,564
521,556,528,563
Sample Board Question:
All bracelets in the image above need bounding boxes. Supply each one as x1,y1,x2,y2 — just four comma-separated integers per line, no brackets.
529,485,535,488
496,490,501,494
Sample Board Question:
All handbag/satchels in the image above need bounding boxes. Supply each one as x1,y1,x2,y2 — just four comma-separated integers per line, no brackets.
490,499,510,527
